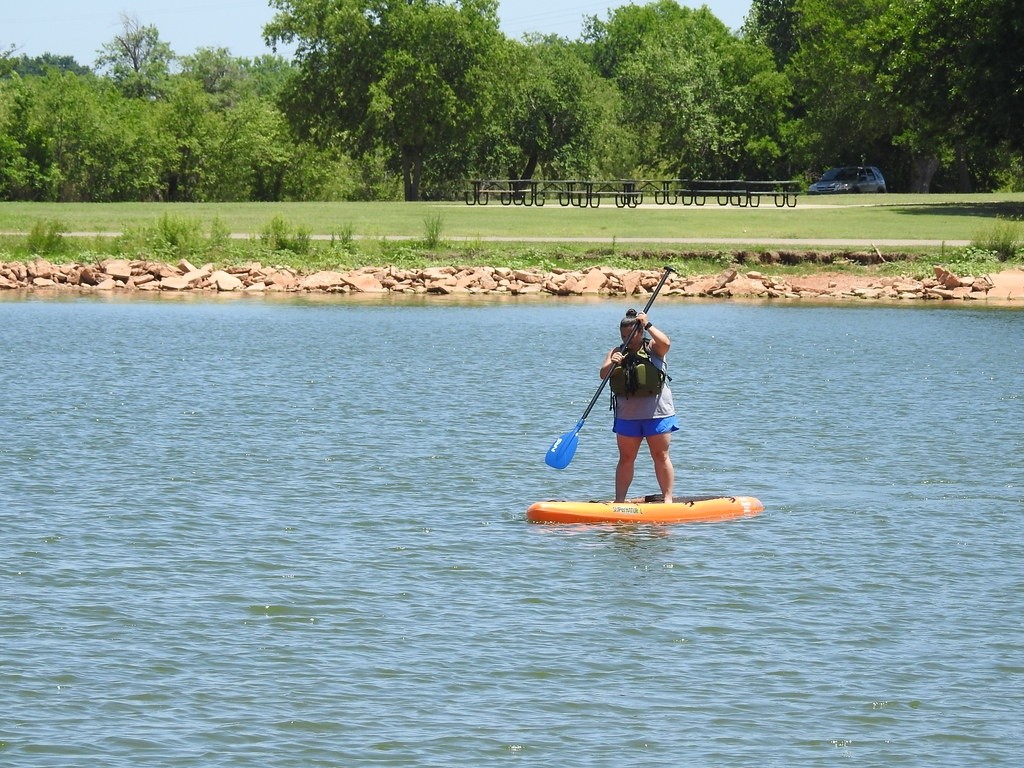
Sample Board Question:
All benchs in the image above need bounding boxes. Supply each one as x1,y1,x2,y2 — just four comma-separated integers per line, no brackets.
464,190,807,208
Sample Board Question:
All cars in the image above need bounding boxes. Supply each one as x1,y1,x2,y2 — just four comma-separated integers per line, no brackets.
807,166,887,195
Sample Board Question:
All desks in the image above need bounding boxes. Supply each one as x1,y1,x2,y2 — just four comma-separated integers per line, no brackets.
470,181,799,208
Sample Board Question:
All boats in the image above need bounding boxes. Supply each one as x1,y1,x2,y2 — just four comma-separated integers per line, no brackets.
526,496,765,526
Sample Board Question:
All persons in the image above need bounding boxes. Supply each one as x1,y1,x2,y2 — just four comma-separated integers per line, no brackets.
600,309,679,503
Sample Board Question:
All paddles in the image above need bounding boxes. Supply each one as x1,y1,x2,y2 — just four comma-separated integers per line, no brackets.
544,265,677,471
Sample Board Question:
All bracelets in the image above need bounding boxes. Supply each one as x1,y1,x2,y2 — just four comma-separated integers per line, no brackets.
645,322,652,330
